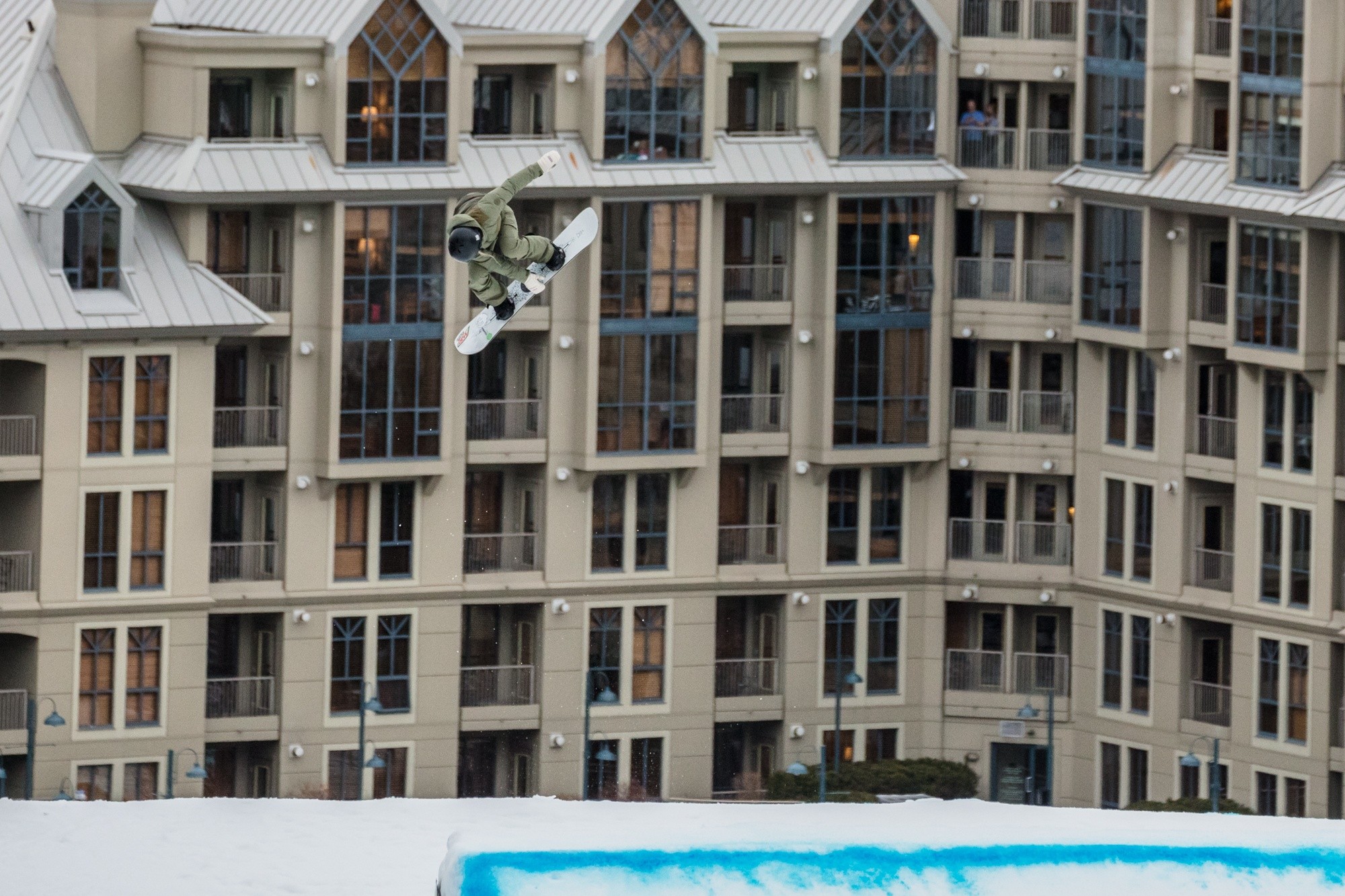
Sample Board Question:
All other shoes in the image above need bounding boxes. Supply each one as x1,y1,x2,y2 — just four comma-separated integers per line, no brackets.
546,247,560,268
494,299,509,317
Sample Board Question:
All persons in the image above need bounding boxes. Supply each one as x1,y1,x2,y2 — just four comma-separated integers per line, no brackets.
960,99,996,166
446,149,566,320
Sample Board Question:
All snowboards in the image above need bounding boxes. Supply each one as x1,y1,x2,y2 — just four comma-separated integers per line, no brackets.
453,206,600,357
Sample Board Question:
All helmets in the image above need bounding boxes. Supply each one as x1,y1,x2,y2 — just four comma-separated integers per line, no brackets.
448,227,480,262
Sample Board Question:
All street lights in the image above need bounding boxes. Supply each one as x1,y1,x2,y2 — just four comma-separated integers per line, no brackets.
164,747,210,800
1017,688,1055,806
1181,735,1221,812
833,671,864,771
356,681,387,800
582,671,620,800
24,696,66,802
787,745,828,803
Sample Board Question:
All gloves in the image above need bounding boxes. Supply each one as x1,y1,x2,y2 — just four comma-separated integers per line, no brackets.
523,272,546,294
537,151,560,173
